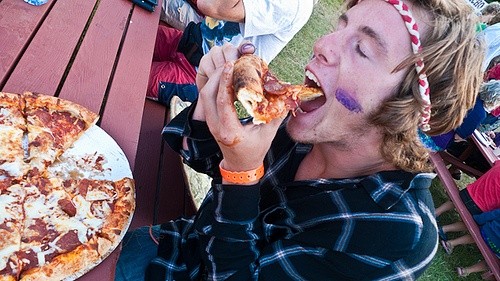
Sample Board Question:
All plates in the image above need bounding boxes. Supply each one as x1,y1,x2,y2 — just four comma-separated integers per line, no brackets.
1,93,136,281
474,129,489,148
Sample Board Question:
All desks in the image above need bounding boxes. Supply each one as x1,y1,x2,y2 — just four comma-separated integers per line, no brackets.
472,129,500,167
0,0,163,281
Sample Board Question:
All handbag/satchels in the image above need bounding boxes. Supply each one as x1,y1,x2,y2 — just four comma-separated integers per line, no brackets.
177,21,204,67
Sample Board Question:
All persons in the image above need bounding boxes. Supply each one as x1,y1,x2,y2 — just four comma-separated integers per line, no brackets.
427,160,500,240
112,0,485,281
420,0,500,153
441,209,500,277
145,0,315,106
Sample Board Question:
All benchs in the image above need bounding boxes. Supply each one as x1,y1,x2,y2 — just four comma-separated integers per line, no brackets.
129,98,194,228
429,152,500,281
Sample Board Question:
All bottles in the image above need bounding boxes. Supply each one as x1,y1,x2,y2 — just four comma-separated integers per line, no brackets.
481,132,497,151
492,145,500,157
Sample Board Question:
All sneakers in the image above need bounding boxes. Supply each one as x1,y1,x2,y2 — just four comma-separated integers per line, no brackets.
439,225,449,240
457,267,469,277
442,239,453,254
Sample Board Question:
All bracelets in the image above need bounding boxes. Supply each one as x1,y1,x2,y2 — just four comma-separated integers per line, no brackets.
216,156,267,187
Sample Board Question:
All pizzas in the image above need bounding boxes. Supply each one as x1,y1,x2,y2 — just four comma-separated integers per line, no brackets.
0,90,135,281
232,53,324,124
